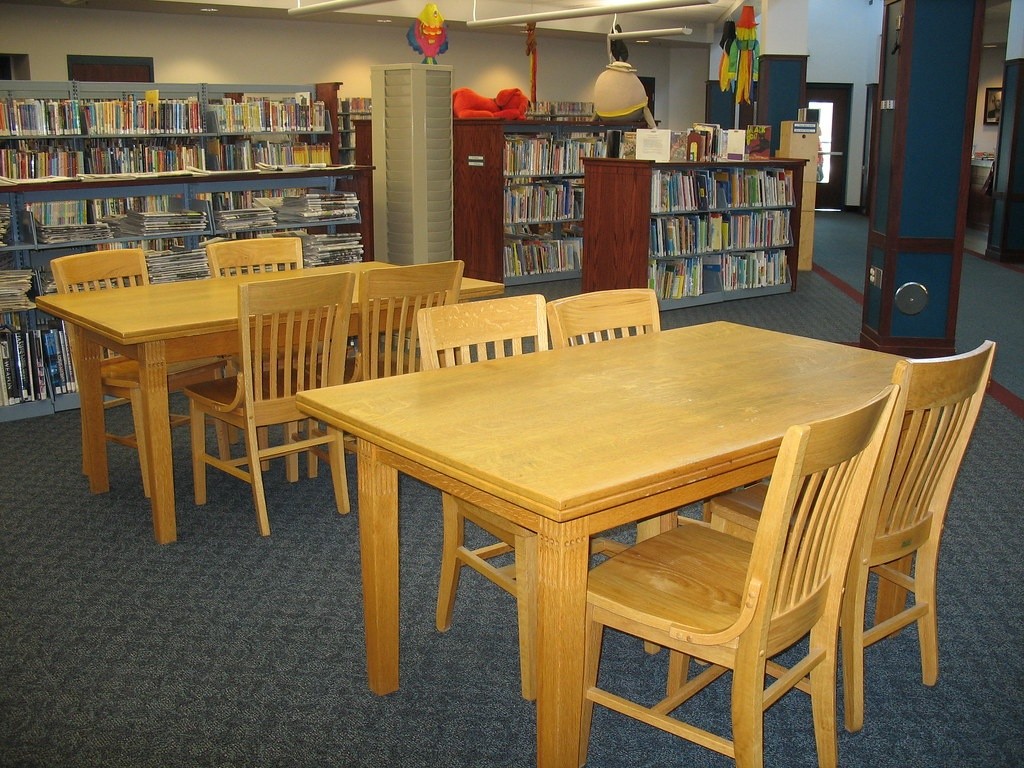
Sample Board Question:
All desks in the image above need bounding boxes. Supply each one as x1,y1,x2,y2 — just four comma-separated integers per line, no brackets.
296,320,913,768
36,261,507,544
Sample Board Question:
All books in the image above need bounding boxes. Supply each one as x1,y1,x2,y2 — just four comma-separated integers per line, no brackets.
1,96,819,408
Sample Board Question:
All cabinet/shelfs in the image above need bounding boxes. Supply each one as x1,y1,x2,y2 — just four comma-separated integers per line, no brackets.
0,79,376,424
577,155,811,310
452,115,661,286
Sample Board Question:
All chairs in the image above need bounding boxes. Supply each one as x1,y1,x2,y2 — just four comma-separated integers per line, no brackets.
182,271,356,536
49,248,228,499
577,383,901,768
716,339,996,735
306,259,466,478
204,235,355,444
410,286,711,700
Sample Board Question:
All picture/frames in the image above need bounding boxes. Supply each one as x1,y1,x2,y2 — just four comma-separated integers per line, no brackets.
983,87,1002,126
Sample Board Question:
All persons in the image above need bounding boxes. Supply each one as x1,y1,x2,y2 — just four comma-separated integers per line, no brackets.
817,126,824,181
988,91,1005,122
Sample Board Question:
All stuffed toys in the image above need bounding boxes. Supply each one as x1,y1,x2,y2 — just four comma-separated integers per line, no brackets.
590,60,656,129
452,87,531,121
